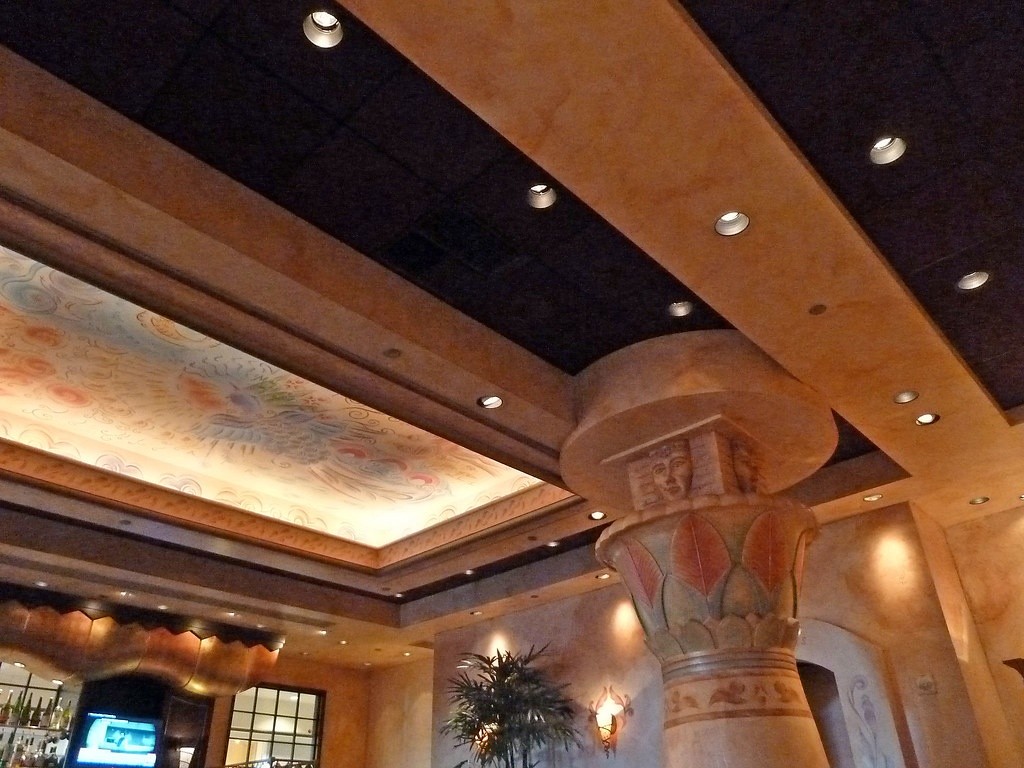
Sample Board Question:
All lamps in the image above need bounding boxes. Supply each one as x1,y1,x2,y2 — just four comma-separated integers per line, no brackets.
596,713,617,758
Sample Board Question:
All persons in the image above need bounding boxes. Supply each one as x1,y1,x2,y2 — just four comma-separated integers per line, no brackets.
648,440,695,505
731,437,759,495
